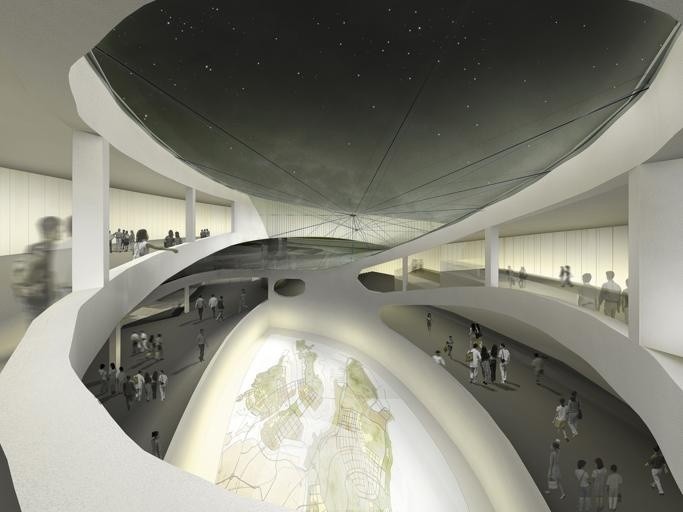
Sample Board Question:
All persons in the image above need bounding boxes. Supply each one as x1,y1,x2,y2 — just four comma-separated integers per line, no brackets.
505,263,528,290
556,264,632,319
95,362,169,410
544,390,625,512
195,328,209,363
108,226,183,259
11,215,72,321
424,311,433,334
194,288,249,321
431,322,545,388
644,446,670,496
128,329,165,361
199,228,210,238
150,430,162,460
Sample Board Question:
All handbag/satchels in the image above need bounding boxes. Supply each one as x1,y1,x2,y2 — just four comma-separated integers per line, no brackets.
576,408,583,419
465,352,473,363
443,346,447,352
547,479,558,490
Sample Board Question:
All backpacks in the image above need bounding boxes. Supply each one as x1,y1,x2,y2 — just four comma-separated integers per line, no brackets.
10,249,47,303
